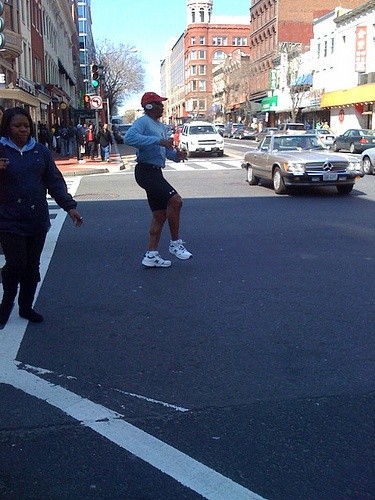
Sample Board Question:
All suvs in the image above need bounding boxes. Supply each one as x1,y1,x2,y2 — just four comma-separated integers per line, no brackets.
178,121,225,157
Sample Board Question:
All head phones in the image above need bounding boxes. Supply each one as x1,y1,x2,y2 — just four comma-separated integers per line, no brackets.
143,104,153,114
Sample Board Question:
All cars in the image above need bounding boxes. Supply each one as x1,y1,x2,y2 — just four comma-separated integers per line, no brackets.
310,129,338,147
332,128,375,155
239,133,364,196
360,147,375,176
213,122,311,143
111,116,182,144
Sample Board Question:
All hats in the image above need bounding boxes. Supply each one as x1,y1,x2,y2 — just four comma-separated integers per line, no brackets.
141,91,167,106
77,124,82,129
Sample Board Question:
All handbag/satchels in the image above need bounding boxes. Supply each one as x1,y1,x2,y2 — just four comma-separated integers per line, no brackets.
80,145,86,153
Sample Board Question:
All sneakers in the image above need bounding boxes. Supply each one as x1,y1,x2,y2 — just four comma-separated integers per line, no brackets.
141,251,171,267
168,240,193,260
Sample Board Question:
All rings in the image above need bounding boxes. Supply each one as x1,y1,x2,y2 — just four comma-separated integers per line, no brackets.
80,217,83,220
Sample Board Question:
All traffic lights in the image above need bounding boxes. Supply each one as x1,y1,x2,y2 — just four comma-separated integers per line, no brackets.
92,64,101,88
83,94,90,103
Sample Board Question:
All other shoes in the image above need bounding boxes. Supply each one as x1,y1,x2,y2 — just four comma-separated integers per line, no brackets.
19,305,45,323
79,160,86,163
0,302,13,329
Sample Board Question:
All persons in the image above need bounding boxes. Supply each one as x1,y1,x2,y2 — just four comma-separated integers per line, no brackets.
321,121,329,130
122,92,192,268
76,124,86,163
0,107,84,328
98,124,112,162
37,121,98,160
